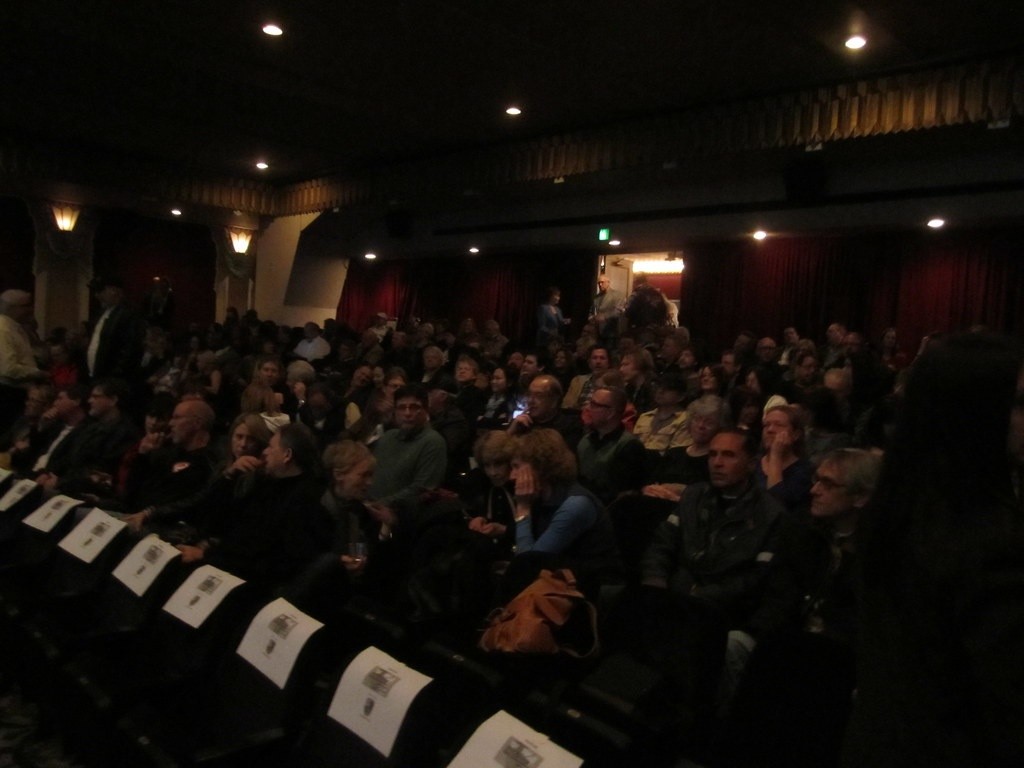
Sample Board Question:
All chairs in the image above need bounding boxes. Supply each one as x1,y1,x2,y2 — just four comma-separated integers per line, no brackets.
0,467,678,768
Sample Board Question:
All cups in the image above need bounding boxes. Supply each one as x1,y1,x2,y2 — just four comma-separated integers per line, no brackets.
349,541,368,562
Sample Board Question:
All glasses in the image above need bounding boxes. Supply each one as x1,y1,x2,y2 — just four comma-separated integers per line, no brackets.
811,474,853,489
590,400,611,410
524,390,552,399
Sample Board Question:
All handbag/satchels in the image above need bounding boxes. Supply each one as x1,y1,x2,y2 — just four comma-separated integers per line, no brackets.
452,467,485,500
477,569,601,659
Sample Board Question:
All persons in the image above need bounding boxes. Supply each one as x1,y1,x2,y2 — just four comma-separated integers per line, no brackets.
83,275,145,379
143,279,178,329
0,286,1024,768
588,275,627,331
625,275,670,334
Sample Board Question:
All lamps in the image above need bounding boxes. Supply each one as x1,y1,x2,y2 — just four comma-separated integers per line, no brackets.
229,225,256,255
52,198,81,233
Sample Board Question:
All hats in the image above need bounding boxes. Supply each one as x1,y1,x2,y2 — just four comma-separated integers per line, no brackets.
651,372,688,392
45,326,67,345
86,273,124,290
